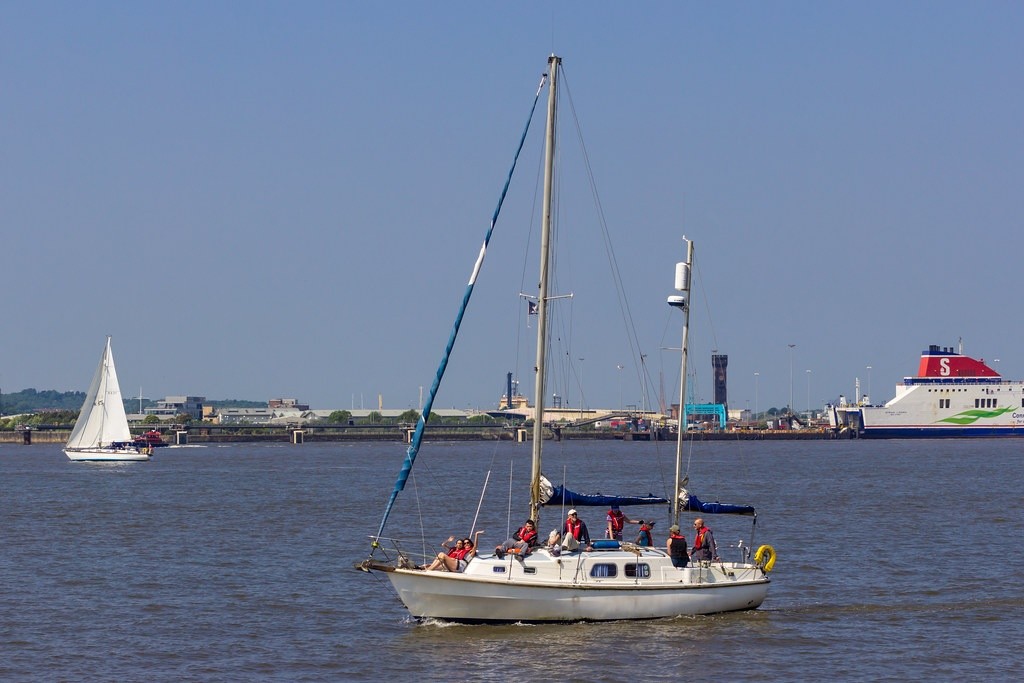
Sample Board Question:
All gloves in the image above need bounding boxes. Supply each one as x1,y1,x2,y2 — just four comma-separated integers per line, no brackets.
638,520,645,524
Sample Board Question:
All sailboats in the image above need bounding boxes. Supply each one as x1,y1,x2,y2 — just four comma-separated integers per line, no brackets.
353,50,777,625
60,334,154,461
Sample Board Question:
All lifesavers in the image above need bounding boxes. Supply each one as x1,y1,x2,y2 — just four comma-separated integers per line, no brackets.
754,544,777,572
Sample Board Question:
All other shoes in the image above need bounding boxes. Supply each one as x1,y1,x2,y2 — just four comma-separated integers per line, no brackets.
515,555,523,562
551,551,560,556
496,548,502,559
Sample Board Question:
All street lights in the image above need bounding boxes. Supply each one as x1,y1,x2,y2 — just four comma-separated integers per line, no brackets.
787,344,797,414
746,399,749,426
753,372,759,421
866,366,871,399
578,359,584,419
805,369,812,419
617,362,624,422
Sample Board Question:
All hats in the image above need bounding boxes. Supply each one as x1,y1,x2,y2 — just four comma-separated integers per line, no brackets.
568,509,577,515
647,519,656,525
610,503,619,510
669,525,680,534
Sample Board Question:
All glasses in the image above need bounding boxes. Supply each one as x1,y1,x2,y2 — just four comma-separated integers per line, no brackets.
694,523,700,526
464,542,470,545
571,513,578,516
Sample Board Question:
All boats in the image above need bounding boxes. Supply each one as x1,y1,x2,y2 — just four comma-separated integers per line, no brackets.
112,430,169,448
858,336,1024,439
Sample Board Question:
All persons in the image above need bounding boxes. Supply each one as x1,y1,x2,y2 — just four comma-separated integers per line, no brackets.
495,519,538,562
667,524,691,568
605,504,644,541
687,518,719,563
634,517,657,546
426,530,485,573
546,509,593,556
425,535,464,570
